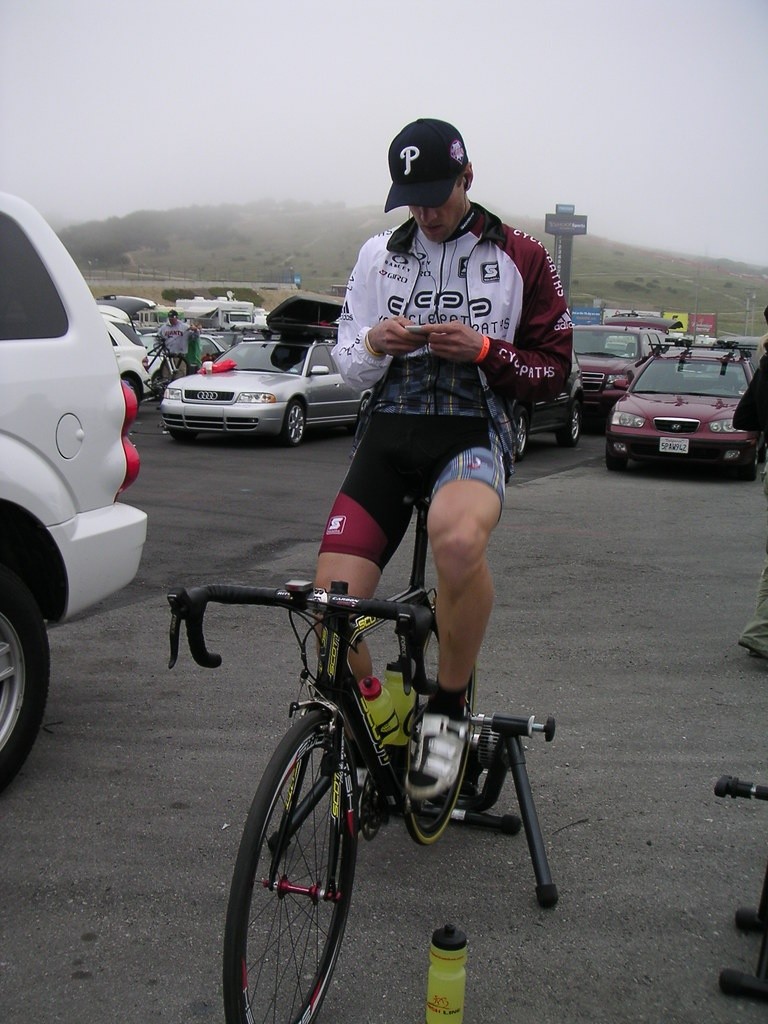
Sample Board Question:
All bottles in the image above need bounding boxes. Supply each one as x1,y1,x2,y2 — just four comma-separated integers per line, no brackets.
384,661,417,747
426,925,466,1024
358,676,401,743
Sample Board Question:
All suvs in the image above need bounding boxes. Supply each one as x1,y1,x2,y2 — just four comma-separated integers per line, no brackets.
570,314,684,435
510,341,584,464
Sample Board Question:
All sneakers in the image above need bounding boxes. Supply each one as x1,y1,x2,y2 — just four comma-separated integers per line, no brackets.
404,697,471,800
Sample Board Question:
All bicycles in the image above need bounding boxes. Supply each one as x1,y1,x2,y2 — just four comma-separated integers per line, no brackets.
166,485,477,1024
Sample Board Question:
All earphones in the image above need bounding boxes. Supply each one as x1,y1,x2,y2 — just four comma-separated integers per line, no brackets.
464,174,469,190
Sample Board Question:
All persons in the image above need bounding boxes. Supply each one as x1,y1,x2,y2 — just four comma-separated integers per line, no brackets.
730,312,768,659
313,120,573,798
152,309,200,410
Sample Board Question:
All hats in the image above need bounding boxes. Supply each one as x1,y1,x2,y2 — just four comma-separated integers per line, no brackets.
384,118,469,214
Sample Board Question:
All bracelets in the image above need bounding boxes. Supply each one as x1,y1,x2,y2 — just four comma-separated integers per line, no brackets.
365,333,385,356
474,334,490,367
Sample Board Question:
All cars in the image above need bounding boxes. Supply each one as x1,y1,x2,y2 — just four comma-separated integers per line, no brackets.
0,194,148,798
160,332,375,449
703,335,768,388
94,290,270,407
606,336,768,482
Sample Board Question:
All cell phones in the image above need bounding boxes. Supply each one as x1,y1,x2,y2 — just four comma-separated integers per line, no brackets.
405,325,428,336
